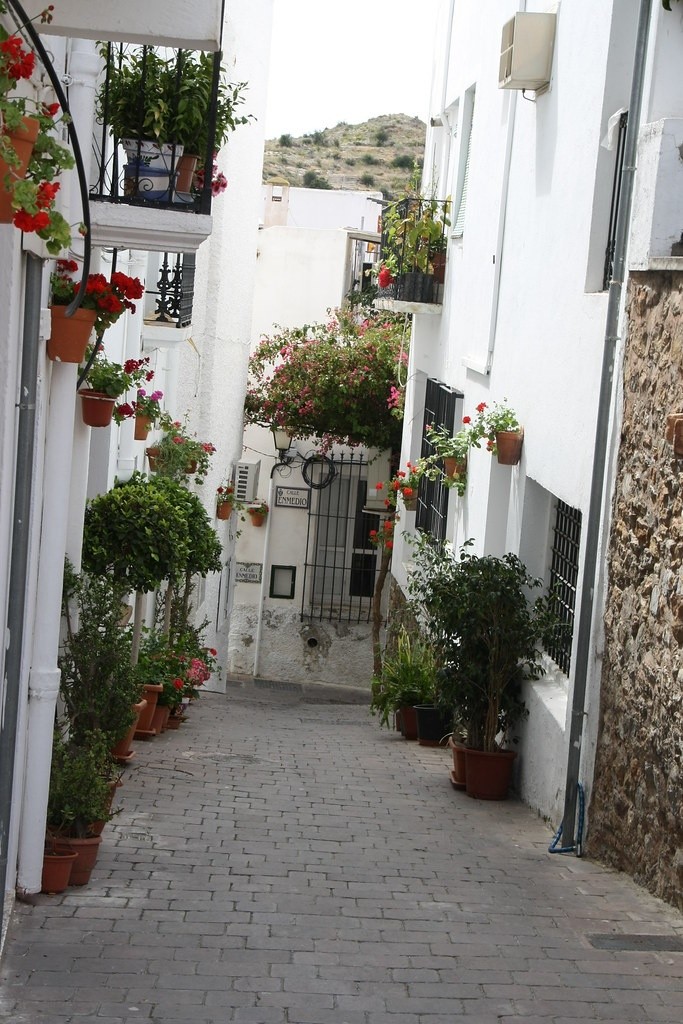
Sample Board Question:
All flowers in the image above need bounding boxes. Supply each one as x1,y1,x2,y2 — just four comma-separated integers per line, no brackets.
192,148,227,197
416,421,471,497
0,0,88,256
375,461,419,506
48,256,145,337
215,478,246,541
246,498,270,522
463,397,518,458
377,260,397,288
152,413,218,487
135,389,164,432
369,514,400,555
141,614,222,708
77,340,155,427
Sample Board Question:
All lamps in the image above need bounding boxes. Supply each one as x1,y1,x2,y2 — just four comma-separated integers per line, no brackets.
271,426,294,463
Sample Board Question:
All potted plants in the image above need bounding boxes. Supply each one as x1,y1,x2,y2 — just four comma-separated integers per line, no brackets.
41,728,127,887
58,551,155,835
172,81,258,194
369,525,573,800
114,469,227,741
81,485,194,764
381,153,454,304
120,153,176,205
94,38,233,171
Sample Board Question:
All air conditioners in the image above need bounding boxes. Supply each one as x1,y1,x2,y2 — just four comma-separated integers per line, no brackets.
497,11,558,91
234,456,262,501
365,444,402,511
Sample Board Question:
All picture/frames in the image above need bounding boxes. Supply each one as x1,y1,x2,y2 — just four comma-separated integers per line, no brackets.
274,486,310,509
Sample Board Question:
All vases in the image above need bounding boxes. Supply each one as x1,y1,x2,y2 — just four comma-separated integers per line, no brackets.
251,511,265,527
42,849,78,894
185,460,196,474
403,487,419,511
77,387,119,427
494,431,524,466
443,457,467,482
149,690,194,737
217,500,233,520
133,414,152,440
146,448,161,471
44,306,97,362
0,110,40,224
